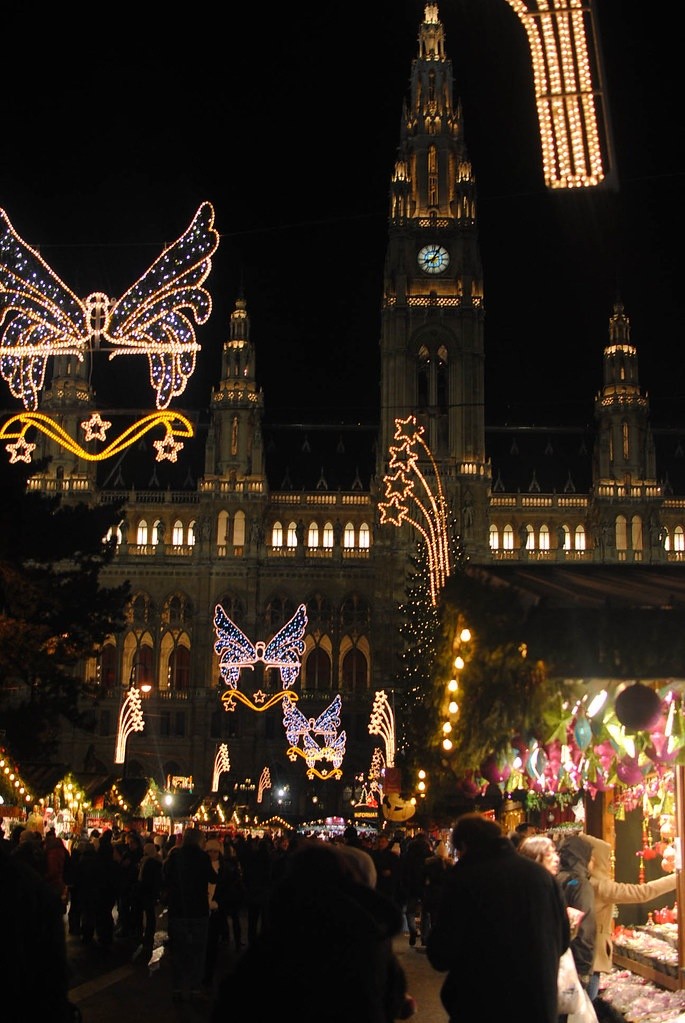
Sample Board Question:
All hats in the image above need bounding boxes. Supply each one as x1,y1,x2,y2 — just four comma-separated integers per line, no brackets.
345,828,357,838
204,839,221,851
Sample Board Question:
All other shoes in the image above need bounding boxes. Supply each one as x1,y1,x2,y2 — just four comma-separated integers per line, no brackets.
409,929,417,947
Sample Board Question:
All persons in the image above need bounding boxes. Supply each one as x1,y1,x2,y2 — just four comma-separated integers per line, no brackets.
519,836,581,940
518,823,535,838
0,817,436,1023
425,816,570,1023
557,832,677,1001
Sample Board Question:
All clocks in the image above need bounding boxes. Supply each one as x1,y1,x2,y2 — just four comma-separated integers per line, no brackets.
417,243,452,279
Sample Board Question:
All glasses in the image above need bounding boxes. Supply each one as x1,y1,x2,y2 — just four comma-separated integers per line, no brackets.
542,850,561,862
